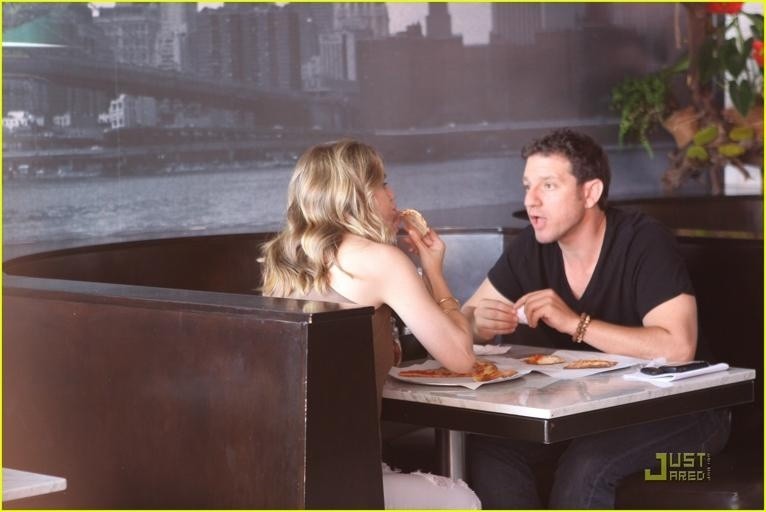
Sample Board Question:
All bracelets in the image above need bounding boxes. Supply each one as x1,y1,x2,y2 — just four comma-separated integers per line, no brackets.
577,314,591,345
437,296,461,306
571,312,587,342
442,305,461,314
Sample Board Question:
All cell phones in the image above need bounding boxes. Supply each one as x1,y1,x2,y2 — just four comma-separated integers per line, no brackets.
659,359,709,373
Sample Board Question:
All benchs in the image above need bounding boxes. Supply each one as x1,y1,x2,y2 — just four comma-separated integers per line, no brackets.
2,193,763,510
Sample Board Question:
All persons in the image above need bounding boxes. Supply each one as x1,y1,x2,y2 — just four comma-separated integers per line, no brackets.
258,136,484,510
461,125,732,510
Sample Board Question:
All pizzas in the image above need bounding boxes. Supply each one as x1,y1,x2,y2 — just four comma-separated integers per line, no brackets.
564,359,619,369
399,359,519,382
523,353,563,365
400,210,430,237
